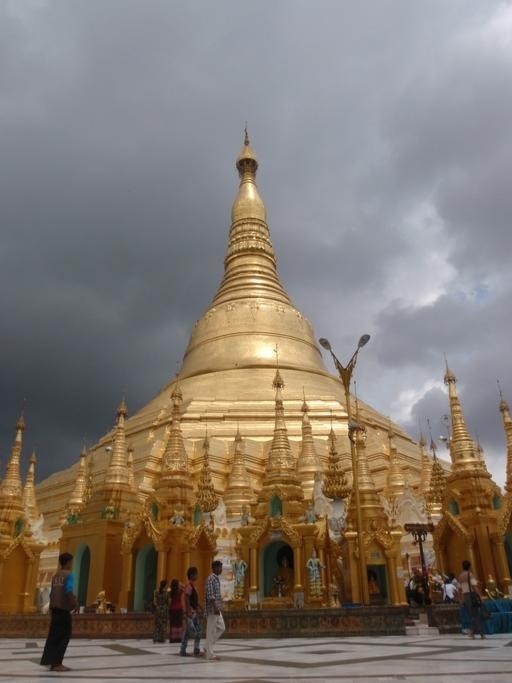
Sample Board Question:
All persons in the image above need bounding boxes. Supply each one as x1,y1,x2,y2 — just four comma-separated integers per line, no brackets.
484,573,512,599
459,560,487,639
271,555,292,598
167,578,185,643
405,559,462,609
40,551,81,672
203,561,225,660
232,556,249,583
306,548,325,581
179,567,205,656
152,581,171,643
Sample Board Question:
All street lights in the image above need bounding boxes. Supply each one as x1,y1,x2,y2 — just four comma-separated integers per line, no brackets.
318,331,372,605
403,522,440,626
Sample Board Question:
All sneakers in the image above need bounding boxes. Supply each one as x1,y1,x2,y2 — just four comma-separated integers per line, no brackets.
50,664,70,671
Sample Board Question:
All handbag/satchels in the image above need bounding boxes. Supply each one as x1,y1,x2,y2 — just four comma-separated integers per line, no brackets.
445,596,451,601
